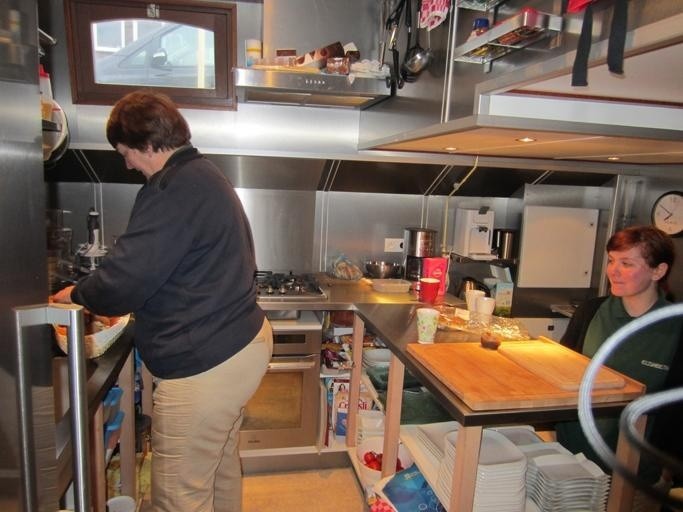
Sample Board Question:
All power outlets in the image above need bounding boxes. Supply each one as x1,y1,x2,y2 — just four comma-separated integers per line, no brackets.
383,237,405,253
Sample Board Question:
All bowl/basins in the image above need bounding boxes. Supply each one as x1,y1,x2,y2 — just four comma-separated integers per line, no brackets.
365,260,400,279
356,437,414,486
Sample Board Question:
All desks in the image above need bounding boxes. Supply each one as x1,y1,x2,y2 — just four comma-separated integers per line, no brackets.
346,304,648,512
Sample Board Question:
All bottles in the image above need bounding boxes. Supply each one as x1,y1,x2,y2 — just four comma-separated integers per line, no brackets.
244,39,297,66
474,18,490,35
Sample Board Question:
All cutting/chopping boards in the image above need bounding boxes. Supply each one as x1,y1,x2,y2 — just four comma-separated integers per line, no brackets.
405,342,646,412
315,272,373,288
498,340,626,391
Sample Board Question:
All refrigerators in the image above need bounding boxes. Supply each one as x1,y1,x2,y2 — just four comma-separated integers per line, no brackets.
0,1,90,512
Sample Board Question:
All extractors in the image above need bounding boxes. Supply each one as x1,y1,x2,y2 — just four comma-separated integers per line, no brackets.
233,0,391,111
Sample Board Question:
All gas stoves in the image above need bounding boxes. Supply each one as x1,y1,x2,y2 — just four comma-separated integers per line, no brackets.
255,270,327,301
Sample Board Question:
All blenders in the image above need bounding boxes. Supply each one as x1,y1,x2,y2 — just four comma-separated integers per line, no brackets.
451,207,498,261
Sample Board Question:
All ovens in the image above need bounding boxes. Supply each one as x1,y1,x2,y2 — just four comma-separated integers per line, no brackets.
236,310,323,450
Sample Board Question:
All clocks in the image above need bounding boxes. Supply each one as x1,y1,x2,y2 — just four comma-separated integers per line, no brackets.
651,190,683,238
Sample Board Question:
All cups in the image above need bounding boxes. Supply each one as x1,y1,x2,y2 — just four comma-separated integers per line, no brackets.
417,307,440,344
419,257,448,304
465,290,496,314
107,496,137,512
492,228,515,260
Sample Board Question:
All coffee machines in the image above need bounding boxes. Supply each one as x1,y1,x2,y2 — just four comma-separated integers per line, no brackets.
402,227,437,280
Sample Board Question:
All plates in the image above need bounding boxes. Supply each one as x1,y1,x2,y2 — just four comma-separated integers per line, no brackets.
362,349,393,366
414,420,611,512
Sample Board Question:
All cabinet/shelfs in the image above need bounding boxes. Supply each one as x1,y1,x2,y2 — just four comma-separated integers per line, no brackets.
454,0,564,73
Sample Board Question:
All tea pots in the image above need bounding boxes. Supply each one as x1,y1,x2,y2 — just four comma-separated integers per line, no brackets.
457,277,490,301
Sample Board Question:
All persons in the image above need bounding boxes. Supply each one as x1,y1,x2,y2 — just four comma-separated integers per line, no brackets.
558,225,683,494
48,92,274,512
338,384,348,392
